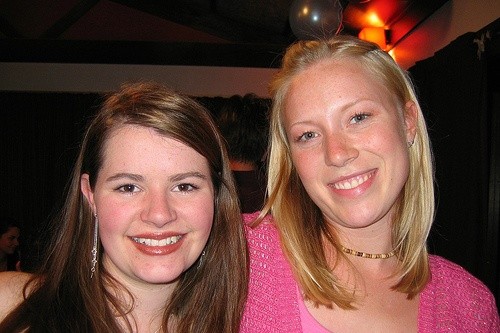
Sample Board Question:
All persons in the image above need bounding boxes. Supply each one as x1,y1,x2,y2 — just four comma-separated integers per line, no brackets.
0,217,22,272
218,102,266,191
236,34,500,333
0,78,250,333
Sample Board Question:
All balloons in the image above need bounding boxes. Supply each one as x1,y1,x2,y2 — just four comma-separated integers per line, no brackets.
288,0,344,40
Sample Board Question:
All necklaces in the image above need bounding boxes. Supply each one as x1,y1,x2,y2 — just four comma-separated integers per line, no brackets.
321,223,401,259
